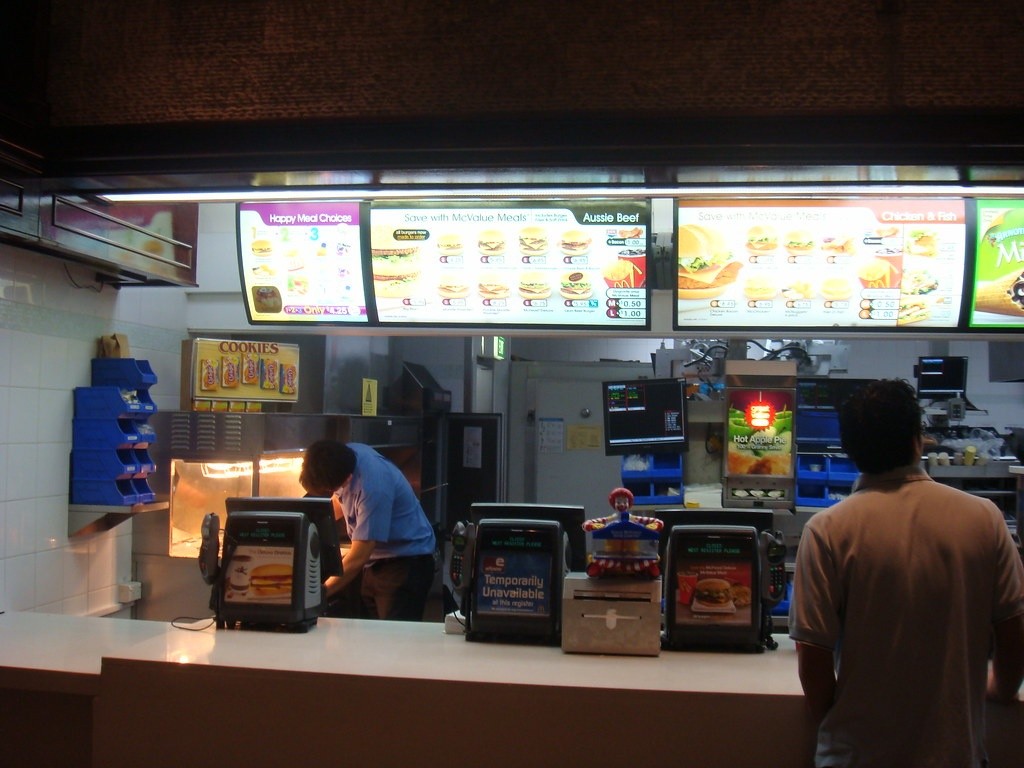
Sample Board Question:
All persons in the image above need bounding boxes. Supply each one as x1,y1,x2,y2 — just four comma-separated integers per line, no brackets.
299,438,436,621
788,377,1024,768
582,487,664,580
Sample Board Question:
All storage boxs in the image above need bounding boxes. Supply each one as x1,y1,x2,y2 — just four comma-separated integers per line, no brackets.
827,481,855,508
72,478,140,507
73,387,144,418
132,478,157,504
652,479,684,505
621,453,652,478
134,449,157,479
72,449,141,479
622,479,653,506
133,419,157,449
72,418,143,449
798,455,828,483
652,451,683,478
90,357,158,390
136,385,159,418
827,458,860,481
794,479,828,507
796,410,842,445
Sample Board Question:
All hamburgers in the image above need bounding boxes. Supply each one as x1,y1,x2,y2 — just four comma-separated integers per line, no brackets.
746,269,852,301
252,240,273,257
434,226,594,300
694,578,732,608
371,223,421,297
745,225,855,255
248,564,293,596
676,225,741,300
899,296,929,325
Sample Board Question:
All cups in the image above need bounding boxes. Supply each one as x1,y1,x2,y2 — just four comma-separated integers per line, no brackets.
231,555,254,602
678,572,697,602
810,464,823,472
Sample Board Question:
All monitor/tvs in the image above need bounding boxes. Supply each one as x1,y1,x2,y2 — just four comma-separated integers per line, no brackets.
472,503,589,572
225,497,344,577
655,508,774,558
602,378,690,456
917,355,968,399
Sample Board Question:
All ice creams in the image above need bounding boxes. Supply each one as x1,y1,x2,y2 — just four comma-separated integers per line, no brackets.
974,269,1024,316
